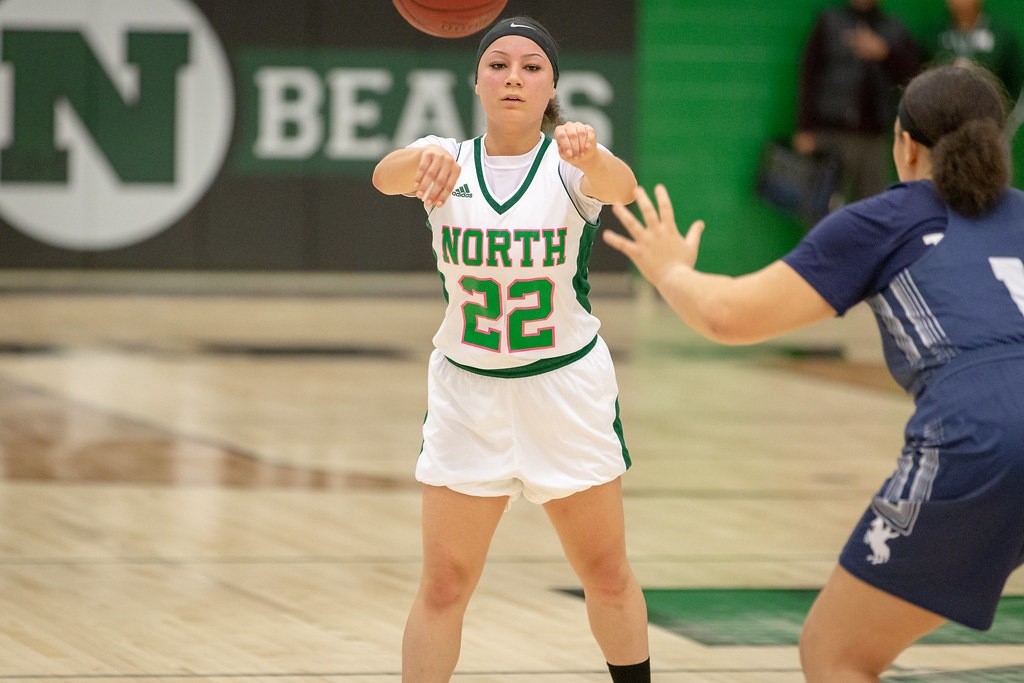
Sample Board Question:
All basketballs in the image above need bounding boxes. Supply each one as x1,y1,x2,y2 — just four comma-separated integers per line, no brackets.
393,0,507,38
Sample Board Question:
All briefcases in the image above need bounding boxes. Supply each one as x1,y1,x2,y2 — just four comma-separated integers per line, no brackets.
760,140,841,220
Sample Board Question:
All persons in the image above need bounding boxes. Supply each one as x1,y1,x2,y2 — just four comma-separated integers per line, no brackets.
372,17,652,683
794,0,1024,235
603,65,1024,683
